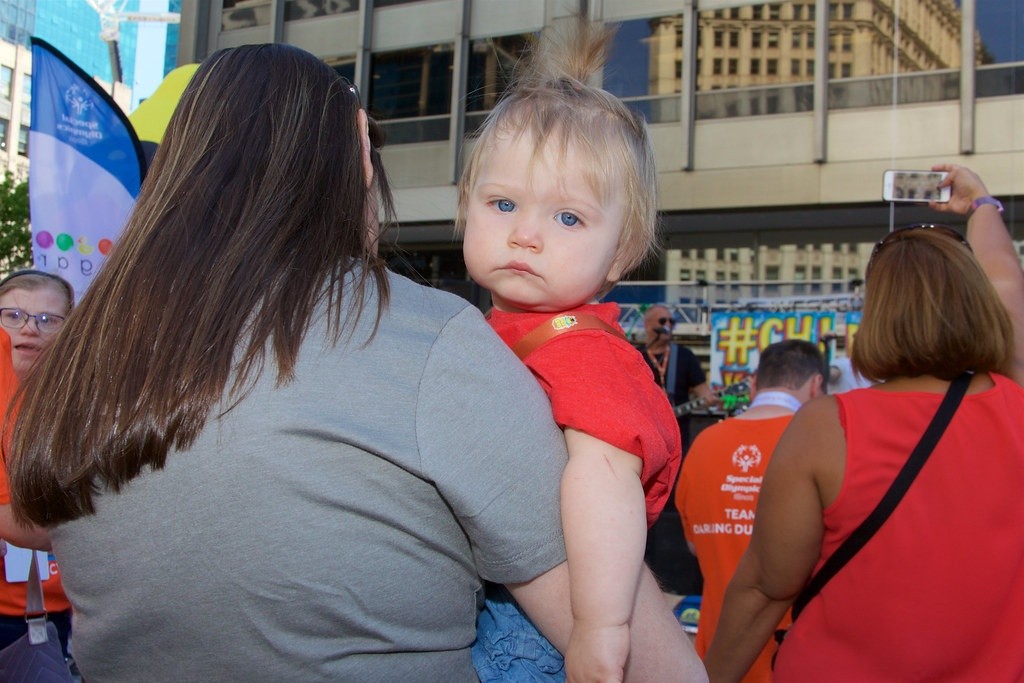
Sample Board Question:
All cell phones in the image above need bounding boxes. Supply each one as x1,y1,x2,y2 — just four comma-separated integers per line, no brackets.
883,170,951,202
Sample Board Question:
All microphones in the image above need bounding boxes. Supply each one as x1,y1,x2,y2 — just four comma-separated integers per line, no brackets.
653,325,671,333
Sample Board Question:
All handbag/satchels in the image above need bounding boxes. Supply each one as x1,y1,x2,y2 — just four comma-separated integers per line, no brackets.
1,546,74,681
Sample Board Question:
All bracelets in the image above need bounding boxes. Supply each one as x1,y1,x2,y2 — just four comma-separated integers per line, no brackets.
965,197,1004,225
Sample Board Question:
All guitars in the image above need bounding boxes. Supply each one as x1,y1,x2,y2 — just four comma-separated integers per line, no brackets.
672,382,751,419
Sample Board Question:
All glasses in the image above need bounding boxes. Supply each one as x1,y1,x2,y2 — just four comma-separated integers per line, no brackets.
0,307,69,336
865,221,972,281
658,317,677,326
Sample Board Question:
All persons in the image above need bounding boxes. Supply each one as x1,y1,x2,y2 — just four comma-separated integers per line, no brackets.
20,46,711,682
460,81,682,683
674,341,829,682
0,271,75,683
707,165,1024,681
631,305,716,511
